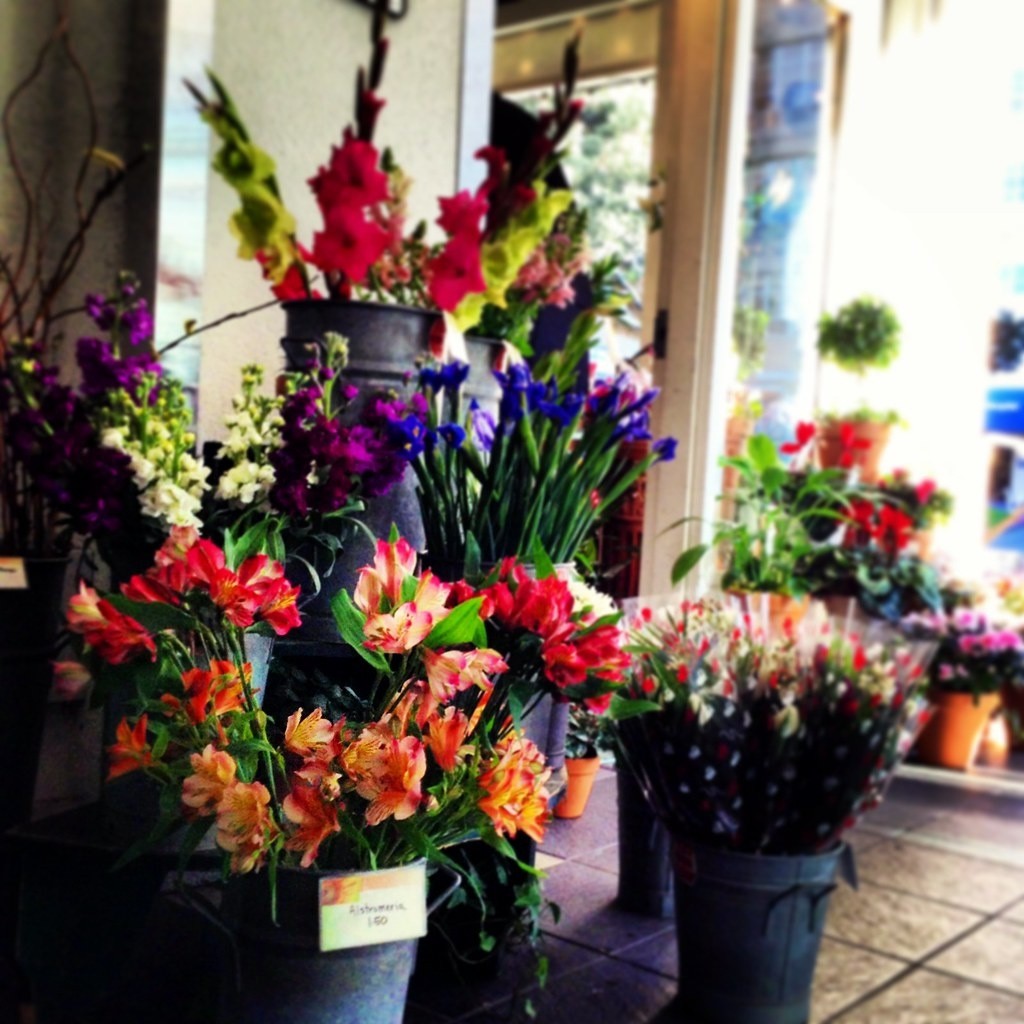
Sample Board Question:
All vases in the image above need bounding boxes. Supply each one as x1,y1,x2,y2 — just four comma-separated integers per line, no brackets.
261,297,440,726
918,686,1003,769
614,763,674,920
219,864,415,1023
0,554,65,865
97,623,273,828
674,834,847,1024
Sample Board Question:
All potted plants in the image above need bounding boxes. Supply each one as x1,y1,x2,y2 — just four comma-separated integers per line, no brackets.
815,301,901,471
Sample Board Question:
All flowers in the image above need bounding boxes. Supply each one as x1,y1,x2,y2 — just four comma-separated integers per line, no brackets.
1,0,1023,1023
